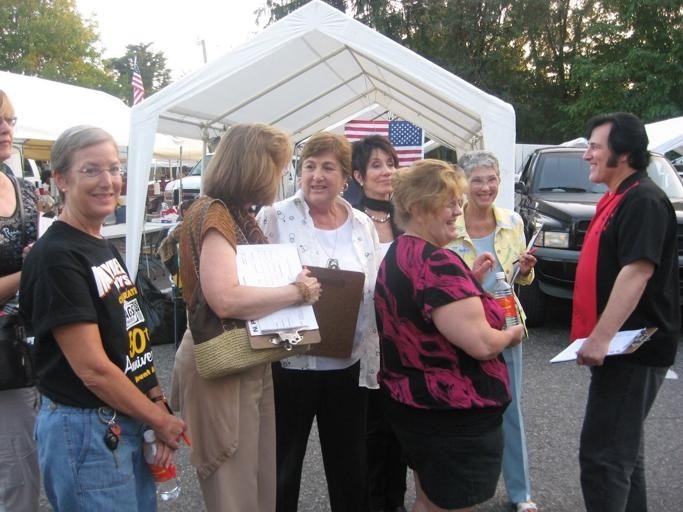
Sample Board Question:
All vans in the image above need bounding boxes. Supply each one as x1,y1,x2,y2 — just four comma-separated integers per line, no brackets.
23,157,50,189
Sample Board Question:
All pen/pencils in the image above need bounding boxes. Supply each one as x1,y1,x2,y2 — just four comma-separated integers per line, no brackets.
163,398,193,448
511,248,538,265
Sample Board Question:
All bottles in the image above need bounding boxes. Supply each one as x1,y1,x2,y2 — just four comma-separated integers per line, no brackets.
142,430,182,501
0,291,20,315
495,271,519,332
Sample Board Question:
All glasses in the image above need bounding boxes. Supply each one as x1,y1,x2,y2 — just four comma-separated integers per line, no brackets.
1,117,18,128
442,199,464,210
1,315,36,391
79,166,127,179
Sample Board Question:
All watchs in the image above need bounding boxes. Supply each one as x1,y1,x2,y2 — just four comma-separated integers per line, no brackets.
151,392,167,405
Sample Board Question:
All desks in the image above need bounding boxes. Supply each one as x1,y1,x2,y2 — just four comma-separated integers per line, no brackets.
98,221,170,281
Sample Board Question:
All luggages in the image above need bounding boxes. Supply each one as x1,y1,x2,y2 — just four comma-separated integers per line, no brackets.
136,239,187,347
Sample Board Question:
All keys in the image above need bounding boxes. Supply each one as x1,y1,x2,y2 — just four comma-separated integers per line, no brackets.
98,405,122,472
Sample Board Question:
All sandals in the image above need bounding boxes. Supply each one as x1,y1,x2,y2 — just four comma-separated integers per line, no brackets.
517,501,538,512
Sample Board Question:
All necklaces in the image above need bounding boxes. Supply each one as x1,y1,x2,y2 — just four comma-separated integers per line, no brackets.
308,203,343,271
363,208,391,223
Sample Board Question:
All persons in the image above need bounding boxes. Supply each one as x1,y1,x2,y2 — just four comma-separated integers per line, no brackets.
371,158,528,512
350,133,402,400
578,113,681,512
445,151,538,512
256,131,382,512
18,122,188,512
173,123,292,511
1,89,41,512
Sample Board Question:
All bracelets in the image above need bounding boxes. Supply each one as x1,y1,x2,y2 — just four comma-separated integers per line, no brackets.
292,280,312,308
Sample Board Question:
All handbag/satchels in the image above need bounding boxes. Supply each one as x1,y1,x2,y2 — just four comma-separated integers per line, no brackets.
187,281,312,383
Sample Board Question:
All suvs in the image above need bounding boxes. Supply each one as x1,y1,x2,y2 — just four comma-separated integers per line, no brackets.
515,148,682,325
164,154,301,216
